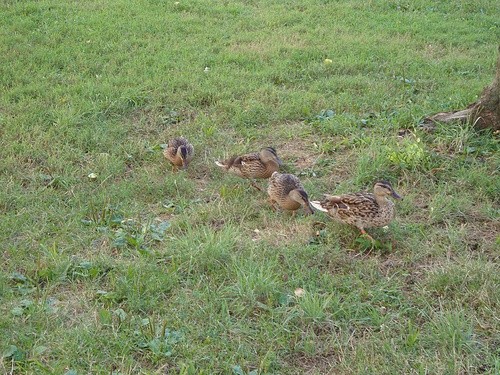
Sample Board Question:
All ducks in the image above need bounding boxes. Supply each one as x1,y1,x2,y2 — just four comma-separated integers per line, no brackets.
307,180,404,245
162,135,194,174
267,170,315,219
213,146,284,194
